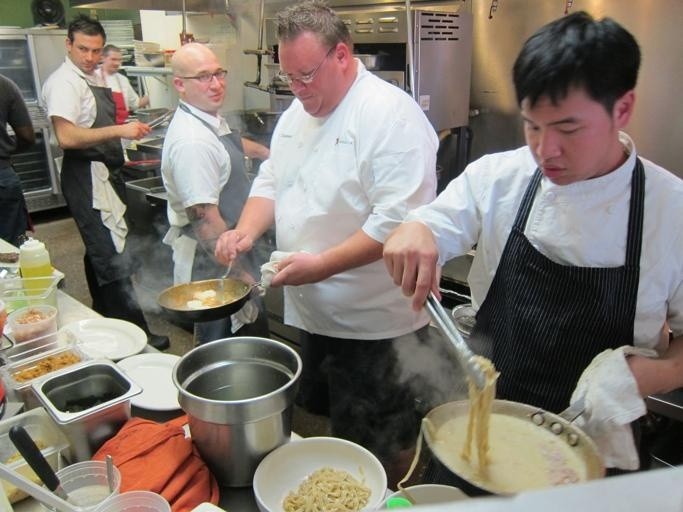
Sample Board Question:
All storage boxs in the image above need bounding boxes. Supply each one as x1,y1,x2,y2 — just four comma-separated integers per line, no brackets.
0,272,91,505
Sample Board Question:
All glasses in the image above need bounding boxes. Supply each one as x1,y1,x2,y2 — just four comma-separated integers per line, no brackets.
177,69,228,82
277,45,336,85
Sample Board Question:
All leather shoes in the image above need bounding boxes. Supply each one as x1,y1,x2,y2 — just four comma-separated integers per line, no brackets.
149,333,170,351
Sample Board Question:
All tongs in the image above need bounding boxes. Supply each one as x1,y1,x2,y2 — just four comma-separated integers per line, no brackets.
423,290,513,395
145,109,175,131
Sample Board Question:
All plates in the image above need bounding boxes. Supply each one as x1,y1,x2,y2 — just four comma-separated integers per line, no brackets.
116,353,179,414
53,316,146,360
130,39,162,67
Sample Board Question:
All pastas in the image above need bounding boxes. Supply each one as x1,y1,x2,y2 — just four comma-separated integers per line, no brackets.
283,465,371,512
398,354,501,505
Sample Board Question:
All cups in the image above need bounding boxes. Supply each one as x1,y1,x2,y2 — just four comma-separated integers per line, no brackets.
38,460,119,511
94,490,171,512
5,304,56,342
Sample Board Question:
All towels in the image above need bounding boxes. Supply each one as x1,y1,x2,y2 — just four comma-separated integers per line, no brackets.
566,345,660,472
169,234,198,287
88,160,127,255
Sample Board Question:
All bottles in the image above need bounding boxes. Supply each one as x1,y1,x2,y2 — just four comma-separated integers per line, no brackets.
162,50,176,67
18,235,58,305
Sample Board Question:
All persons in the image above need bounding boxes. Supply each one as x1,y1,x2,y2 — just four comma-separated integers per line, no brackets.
0,72,36,245
380,9,682,495
215,1,438,478
92,44,150,125
41,12,171,352
161,41,270,348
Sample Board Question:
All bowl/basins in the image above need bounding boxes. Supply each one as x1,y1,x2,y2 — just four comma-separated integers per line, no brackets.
252,436,387,512
375,483,469,510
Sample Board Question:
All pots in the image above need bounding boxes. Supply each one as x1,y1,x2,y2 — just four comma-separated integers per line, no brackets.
158,277,263,320
421,398,604,494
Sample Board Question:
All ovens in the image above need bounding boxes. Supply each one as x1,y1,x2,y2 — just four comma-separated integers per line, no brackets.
264,3,471,132
